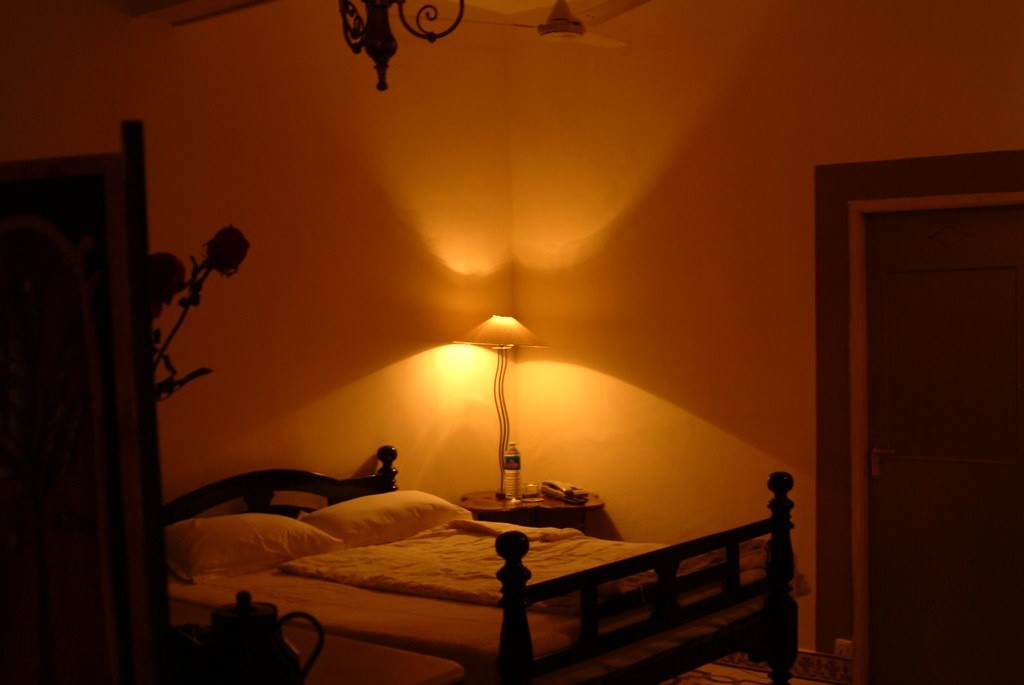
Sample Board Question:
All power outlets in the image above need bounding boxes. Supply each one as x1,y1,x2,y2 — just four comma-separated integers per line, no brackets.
835,639,853,660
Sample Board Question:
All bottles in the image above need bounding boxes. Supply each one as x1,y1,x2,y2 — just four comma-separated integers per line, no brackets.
503,442,521,499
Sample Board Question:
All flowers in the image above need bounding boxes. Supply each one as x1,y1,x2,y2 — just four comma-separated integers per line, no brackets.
136,226,249,424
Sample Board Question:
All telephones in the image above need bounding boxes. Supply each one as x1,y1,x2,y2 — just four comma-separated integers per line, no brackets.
541,480,589,504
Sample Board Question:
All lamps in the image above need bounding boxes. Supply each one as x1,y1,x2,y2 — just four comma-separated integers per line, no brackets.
454,317,549,495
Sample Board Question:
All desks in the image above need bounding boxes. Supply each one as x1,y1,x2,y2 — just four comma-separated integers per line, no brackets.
170,601,465,684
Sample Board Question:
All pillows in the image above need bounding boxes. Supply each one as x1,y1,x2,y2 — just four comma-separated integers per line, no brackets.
302,490,471,547
166,513,343,585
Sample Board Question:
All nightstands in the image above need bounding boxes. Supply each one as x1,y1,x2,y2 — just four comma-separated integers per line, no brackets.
459,491,604,530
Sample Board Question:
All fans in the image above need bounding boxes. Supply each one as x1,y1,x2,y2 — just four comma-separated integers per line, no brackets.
511,1,646,50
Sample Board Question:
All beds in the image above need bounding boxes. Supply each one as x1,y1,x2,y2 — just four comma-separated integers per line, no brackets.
162,445,798,685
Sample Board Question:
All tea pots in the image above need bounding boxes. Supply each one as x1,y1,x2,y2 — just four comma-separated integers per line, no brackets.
172,592,324,685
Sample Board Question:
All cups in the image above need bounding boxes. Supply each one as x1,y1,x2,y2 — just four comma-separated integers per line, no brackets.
524,482,540,500
